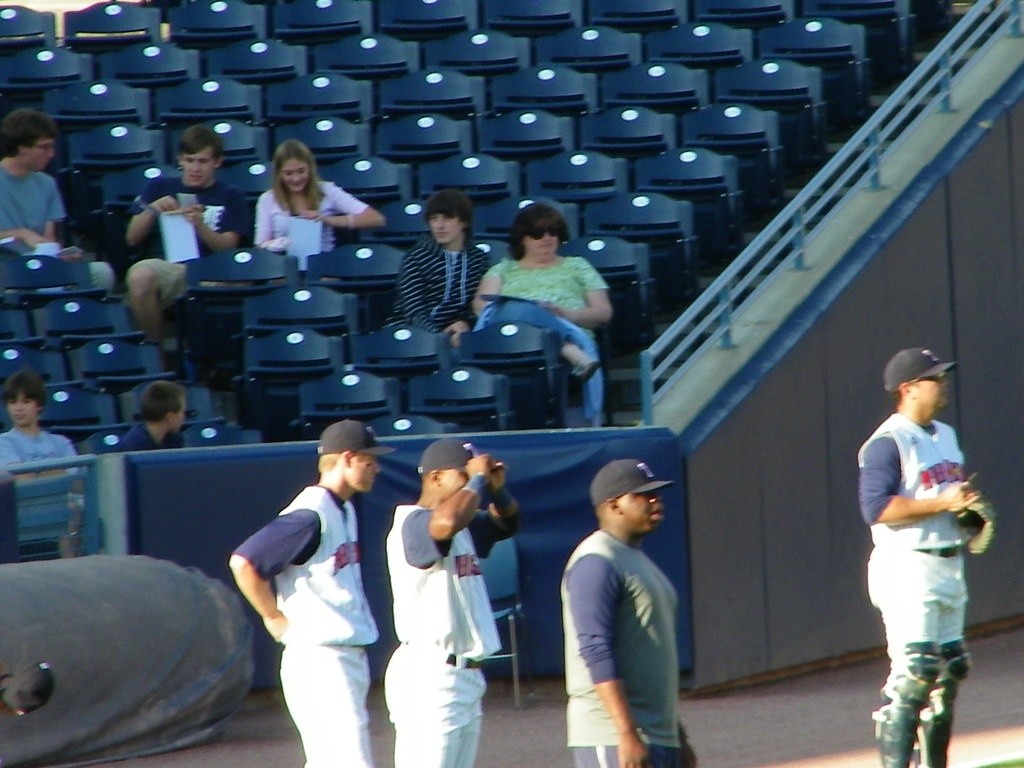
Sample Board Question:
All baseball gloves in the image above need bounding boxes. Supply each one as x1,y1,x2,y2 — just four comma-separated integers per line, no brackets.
956,499,999,555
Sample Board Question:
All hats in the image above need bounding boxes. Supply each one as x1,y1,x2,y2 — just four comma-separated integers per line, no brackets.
318,419,397,455
882,347,957,393
590,459,674,507
418,438,499,474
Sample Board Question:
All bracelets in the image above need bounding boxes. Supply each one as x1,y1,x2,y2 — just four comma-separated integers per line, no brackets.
348,215,354,229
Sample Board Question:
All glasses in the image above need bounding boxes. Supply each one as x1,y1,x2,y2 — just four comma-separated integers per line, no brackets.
25,143,54,151
919,371,947,384
524,227,559,240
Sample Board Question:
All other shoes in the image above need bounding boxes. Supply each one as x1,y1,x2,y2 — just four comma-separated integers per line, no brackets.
573,358,601,381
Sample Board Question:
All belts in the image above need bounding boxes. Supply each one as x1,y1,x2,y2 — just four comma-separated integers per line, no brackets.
920,546,958,557
448,655,479,670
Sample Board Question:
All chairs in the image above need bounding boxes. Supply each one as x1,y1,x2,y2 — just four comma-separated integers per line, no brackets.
476,539,533,710
0,0,955,453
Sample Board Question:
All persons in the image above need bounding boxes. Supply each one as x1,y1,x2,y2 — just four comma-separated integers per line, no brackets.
229,419,396,768
858,348,982,768
254,139,386,267
384,438,522,768
0,109,114,297
382,190,491,343
112,382,185,453
0,370,78,471
561,459,696,768
472,203,612,431
126,125,250,342
